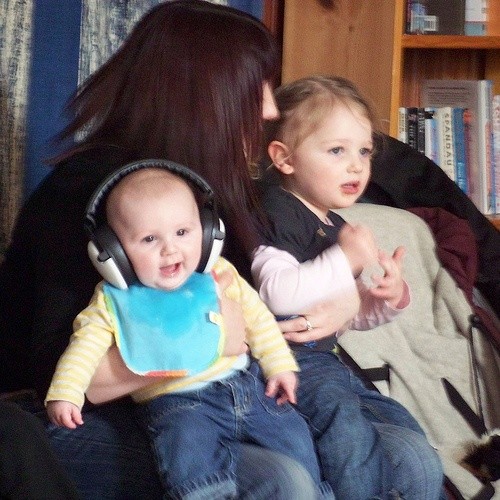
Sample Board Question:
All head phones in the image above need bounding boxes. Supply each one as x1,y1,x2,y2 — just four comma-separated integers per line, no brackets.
84,159,226,291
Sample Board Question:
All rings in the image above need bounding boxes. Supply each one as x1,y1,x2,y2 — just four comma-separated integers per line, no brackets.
304,319,313,331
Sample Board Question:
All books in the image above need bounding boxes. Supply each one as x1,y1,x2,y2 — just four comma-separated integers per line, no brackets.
396,78,500,216
405,0,490,37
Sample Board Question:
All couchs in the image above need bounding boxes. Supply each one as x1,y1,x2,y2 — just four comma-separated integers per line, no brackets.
0,130,500,500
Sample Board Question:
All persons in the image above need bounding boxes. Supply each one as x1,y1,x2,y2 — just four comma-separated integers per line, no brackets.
0,0,280,499
45,158,335,500
247,74,445,500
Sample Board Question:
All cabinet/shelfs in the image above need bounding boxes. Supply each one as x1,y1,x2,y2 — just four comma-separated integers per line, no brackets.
281,0,500,227
